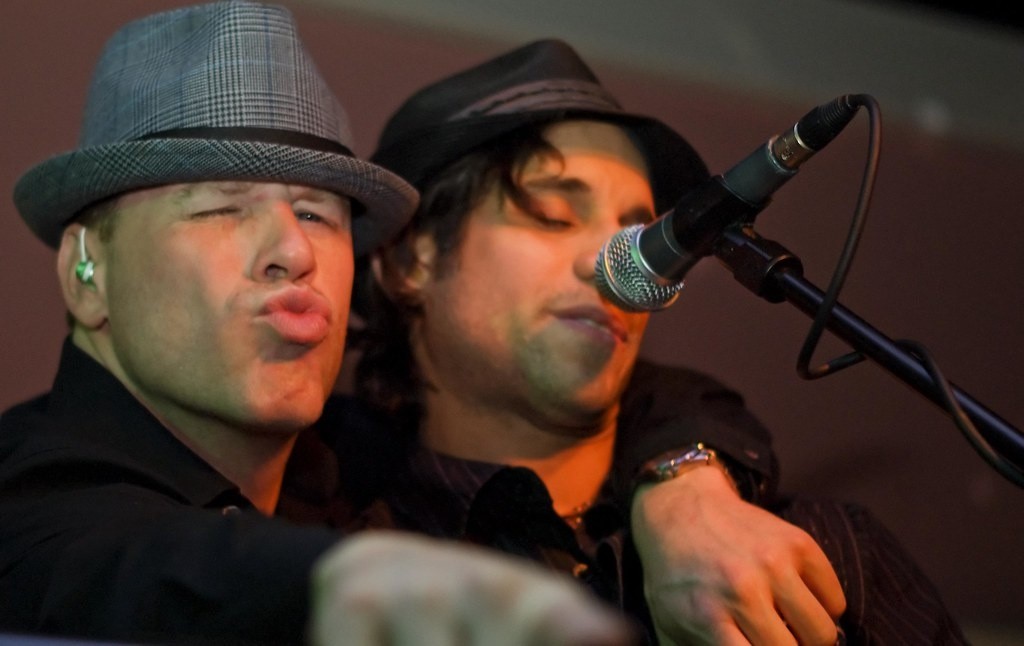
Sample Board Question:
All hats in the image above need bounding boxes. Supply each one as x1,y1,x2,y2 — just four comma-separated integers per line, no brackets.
351,38,712,325
14,0,419,264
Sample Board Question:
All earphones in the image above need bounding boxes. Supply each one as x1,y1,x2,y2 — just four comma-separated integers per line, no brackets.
76,259,97,287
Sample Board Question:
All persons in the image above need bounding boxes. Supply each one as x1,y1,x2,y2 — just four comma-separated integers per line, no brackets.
341,37,970,646
1,0,843,646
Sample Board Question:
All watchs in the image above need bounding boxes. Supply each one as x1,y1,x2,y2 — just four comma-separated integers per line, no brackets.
641,443,744,489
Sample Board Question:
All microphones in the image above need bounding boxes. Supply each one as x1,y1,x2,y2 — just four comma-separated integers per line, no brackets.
593,96,860,313
